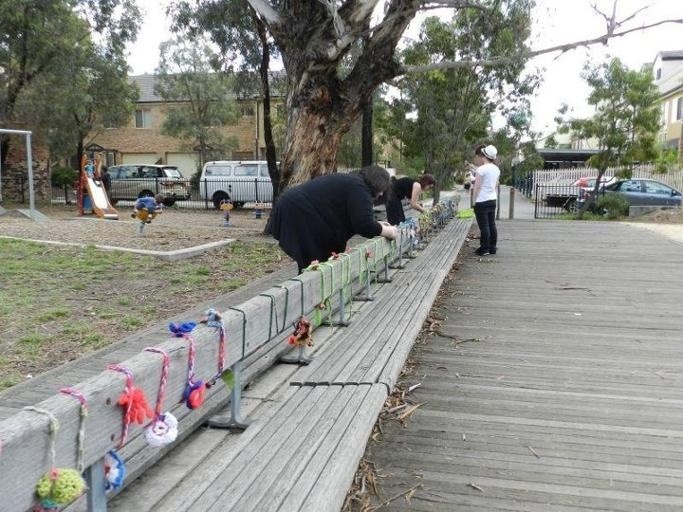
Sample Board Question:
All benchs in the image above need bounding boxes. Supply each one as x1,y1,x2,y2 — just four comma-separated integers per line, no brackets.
0,195,476,512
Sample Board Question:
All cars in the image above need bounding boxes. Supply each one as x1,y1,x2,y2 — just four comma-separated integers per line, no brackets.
545,178,683,217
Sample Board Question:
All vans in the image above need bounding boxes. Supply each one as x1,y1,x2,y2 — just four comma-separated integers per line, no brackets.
107,164,190,207
199,160,281,209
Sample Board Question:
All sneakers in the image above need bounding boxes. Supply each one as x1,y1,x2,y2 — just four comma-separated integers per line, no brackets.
473,246,496,256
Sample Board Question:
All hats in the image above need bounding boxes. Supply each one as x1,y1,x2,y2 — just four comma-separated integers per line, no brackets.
480,144,498,160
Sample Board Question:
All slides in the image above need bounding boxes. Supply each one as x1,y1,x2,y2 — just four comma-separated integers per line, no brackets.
82,171,121,220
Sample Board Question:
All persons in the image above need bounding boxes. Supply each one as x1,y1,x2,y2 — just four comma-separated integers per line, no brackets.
471,144,502,256
386,172,435,226
92,165,114,207
265,164,402,273
84,159,96,178
134,194,163,224
460,145,488,169
